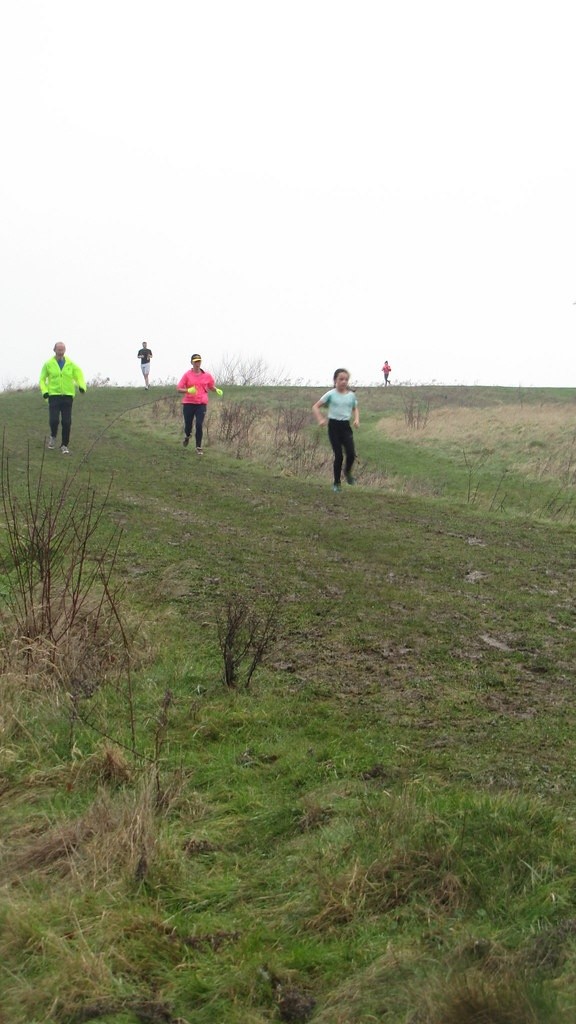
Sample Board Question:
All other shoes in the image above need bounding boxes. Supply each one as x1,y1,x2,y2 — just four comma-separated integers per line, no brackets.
60,446,70,454
183,435,191,446
48,436,57,450
332,482,341,491
196,446,203,455
346,478,353,485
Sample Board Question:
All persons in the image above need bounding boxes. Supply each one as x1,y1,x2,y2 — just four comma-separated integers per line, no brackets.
382,361,391,387
312,369,359,492
177,354,223,455
138,342,152,390
39,342,87,454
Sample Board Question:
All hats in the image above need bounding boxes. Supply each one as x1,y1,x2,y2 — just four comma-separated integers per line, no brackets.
191,354,201,361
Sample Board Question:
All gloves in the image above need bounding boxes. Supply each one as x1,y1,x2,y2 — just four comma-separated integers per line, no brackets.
79,387,84,393
188,385,198,394
216,389,223,397
43,393,49,399
